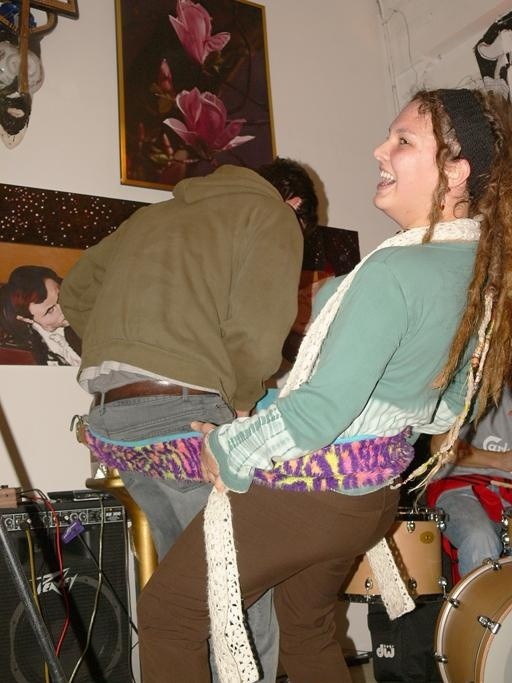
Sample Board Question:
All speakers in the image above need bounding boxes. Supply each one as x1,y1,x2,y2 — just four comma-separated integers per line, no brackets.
0,495,134,682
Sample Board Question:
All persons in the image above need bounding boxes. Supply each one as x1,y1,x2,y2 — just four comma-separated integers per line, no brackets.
6,263,101,366
424,379,510,574
60,156,322,683
137,87,510,683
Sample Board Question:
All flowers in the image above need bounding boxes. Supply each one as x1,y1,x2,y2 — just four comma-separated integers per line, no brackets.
154,0,262,182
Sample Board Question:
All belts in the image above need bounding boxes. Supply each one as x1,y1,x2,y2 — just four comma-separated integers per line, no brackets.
89,378,219,409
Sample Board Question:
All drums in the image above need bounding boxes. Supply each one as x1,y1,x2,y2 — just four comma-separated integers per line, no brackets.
434,556,512,683
339,506,448,604
499,506,512,554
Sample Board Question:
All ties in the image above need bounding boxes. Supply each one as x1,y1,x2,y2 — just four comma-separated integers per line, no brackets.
50,333,82,366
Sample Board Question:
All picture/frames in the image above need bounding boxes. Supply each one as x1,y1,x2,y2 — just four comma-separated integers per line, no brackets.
113,0,280,194
